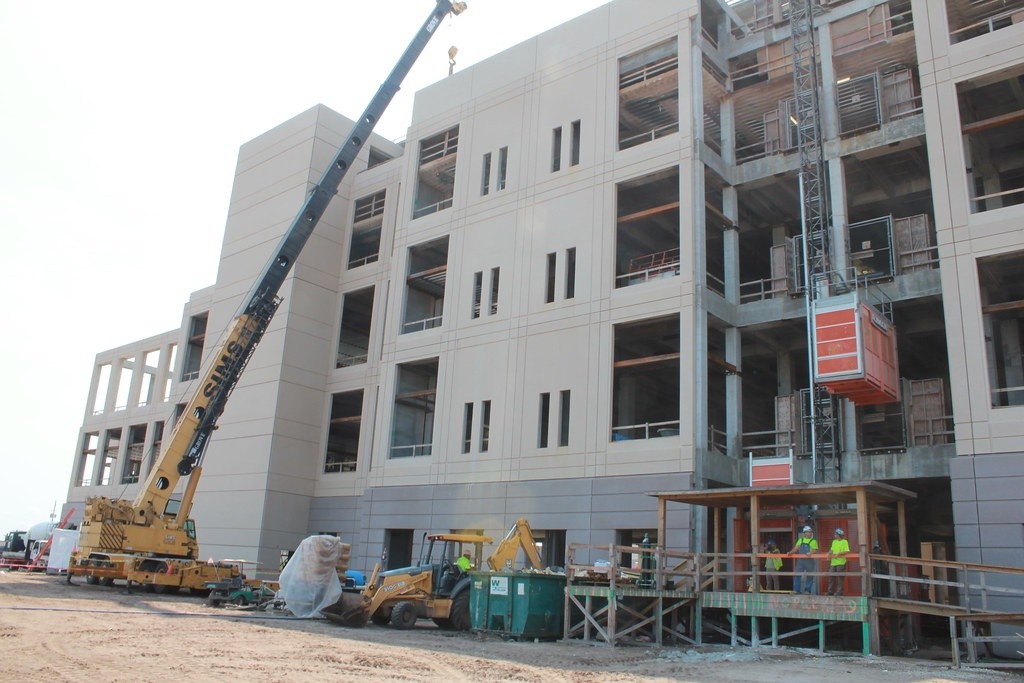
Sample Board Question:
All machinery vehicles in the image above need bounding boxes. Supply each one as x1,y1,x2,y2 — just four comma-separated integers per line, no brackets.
205,558,268,610
318,518,544,631
0,531,31,558
64,0,467,595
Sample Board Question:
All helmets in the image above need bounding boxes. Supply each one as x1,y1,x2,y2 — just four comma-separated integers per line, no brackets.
803,526,812,532
768,540,776,544
463,550,471,555
835,528,844,536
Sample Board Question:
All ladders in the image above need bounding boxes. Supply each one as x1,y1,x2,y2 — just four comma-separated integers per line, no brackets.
26,508,76,572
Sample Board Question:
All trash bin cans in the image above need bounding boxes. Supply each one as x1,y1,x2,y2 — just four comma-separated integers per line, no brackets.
469,570,568,638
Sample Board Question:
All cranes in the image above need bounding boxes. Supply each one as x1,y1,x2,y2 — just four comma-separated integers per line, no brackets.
789,0,846,510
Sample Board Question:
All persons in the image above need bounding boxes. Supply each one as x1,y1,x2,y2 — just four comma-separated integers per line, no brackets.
764,545,783,590
826,529,850,596
457,550,472,572
787,525,818,594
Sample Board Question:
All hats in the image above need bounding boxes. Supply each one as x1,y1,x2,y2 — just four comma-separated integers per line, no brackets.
874,540,879,548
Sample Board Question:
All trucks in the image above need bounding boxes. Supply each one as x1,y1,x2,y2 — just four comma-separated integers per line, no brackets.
2,539,51,571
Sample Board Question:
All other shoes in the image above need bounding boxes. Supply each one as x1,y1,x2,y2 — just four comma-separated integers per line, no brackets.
834,591,843,596
793,591,800,594
804,591,811,594
825,592,833,596
